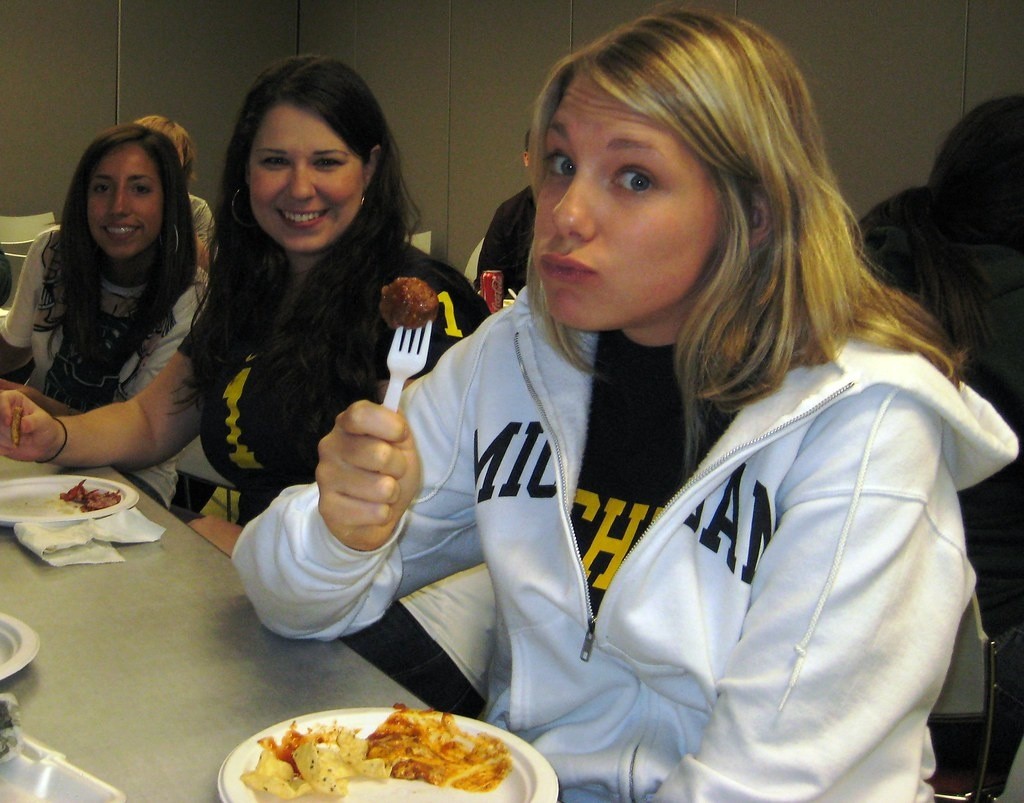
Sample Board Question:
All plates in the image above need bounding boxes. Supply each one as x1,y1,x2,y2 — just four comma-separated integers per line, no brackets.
216,707,561,803
0,612,41,681
0,474,140,530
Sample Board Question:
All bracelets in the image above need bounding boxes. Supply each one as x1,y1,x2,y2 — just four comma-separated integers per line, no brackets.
34,417,67,463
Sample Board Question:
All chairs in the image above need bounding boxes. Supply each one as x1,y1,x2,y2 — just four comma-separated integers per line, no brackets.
0,212,57,321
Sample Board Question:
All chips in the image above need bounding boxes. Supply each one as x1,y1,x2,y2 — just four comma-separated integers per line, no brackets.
238,729,395,800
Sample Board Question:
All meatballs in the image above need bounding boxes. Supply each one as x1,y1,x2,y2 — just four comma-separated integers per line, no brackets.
379,276,439,328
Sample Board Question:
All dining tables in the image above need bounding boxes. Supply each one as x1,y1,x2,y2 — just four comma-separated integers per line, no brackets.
0,456,432,803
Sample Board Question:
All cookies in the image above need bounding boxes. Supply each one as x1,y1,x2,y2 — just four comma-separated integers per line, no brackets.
11,406,23,449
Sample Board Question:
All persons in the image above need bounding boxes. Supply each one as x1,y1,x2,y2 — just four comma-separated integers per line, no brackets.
0,54,492,720
0,123,217,515
472,126,538,302
0,248,12,305
135,116,218,272
860,94,1024,739
232,8,1020,803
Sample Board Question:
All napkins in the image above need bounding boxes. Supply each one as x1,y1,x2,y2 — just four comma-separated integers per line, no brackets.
13,507,167,567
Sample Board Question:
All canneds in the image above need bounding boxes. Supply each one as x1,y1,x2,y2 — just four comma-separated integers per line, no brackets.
481,269,504,314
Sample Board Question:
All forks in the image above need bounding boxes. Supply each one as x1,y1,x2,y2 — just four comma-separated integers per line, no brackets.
383,322,437,413
